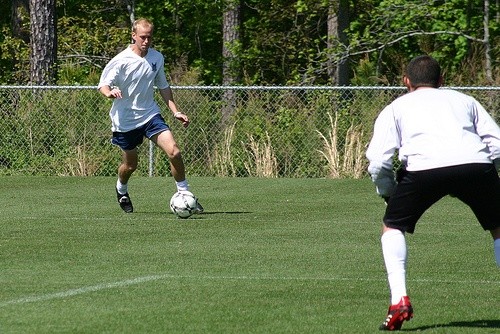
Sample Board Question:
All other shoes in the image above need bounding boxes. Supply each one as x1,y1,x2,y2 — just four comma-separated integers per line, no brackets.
195,201,205,216
114,187,134,213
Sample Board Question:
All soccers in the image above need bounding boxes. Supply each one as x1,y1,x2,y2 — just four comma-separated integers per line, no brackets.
169,191,197,219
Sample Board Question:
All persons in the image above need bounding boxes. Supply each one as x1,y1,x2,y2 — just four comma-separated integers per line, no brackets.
97,16,204,215
365,56,500,330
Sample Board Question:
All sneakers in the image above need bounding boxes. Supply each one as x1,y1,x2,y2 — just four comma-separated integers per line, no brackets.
379,295,415,330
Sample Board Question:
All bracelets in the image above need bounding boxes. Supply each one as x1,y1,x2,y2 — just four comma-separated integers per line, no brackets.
174,112,182,117
